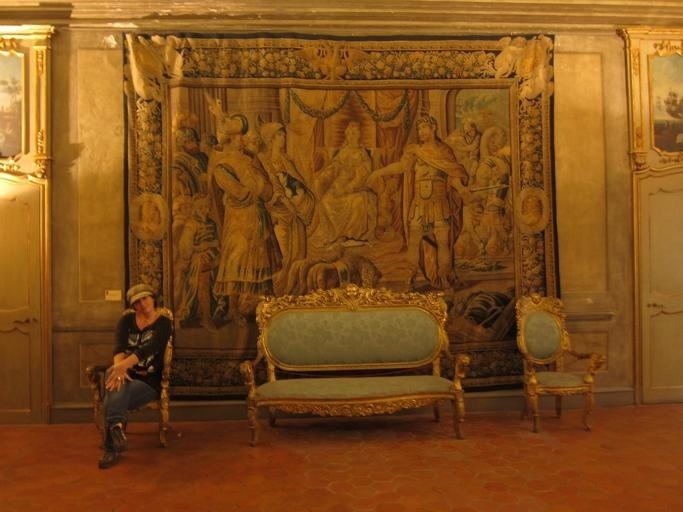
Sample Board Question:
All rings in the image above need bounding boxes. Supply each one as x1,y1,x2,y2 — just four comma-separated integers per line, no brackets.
118,376,120,380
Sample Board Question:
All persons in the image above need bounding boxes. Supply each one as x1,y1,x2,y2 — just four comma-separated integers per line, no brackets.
99,284,173,469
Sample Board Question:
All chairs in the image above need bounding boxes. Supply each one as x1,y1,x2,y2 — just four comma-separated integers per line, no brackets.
85,308,175,448
514,293,602,433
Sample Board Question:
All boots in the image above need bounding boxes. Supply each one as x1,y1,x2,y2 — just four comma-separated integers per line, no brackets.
97,423,128,469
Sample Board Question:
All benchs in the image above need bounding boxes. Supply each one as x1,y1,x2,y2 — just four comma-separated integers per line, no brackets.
240,286,468,447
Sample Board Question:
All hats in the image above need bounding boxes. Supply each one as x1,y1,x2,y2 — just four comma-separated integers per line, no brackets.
126,284,153,306
